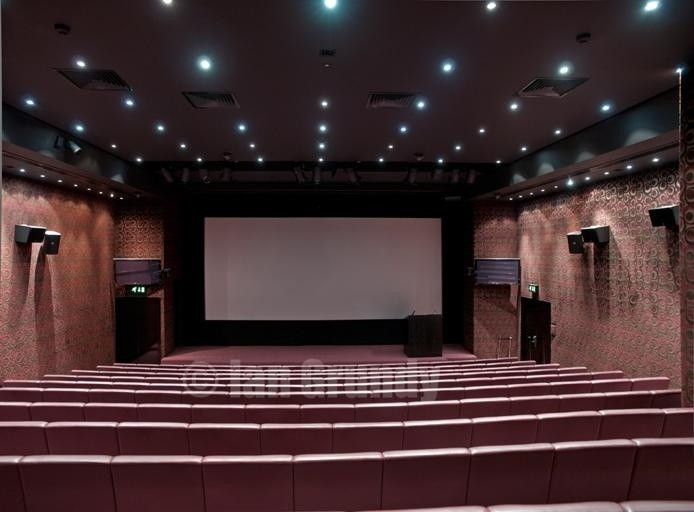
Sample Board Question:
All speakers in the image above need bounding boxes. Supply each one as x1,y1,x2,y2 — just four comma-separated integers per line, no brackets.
567,231,583,253
44,231,61,254
